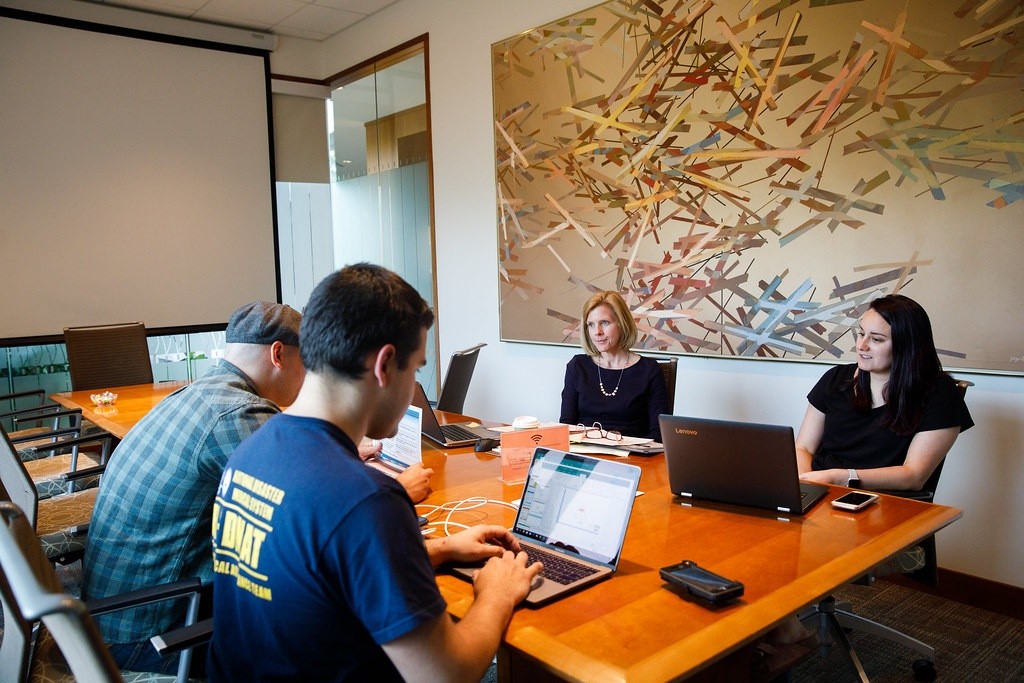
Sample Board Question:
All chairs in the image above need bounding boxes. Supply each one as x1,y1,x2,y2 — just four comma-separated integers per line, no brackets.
751,379,974,683
0,322,213,683
655,357,679,415
435,342,487,415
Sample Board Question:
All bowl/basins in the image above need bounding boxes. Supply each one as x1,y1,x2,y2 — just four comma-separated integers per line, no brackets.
90,394,118,407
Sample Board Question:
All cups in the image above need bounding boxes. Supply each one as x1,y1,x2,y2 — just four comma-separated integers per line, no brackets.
512,416,540,431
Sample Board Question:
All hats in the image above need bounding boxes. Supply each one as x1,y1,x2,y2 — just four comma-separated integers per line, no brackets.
225,300,303,345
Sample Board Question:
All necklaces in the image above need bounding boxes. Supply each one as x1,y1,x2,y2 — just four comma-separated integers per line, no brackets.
598,352,629,396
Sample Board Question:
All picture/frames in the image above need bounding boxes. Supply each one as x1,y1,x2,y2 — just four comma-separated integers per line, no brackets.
491,0,1024,378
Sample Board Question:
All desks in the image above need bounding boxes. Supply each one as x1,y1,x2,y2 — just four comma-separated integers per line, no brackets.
49,379,963,683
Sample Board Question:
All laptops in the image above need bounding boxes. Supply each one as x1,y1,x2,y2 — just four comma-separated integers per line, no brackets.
441,447,642,607
658,414,829,515
410,380,500,447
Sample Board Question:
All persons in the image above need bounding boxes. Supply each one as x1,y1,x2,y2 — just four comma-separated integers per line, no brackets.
80,301,433,683
487,423,664,456
559,291,668,443
753,294,975,683
203,263,544,683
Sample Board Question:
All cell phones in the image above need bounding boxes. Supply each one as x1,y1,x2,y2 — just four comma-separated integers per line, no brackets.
659,561,744,602
417,515,429,527
831,491,880,510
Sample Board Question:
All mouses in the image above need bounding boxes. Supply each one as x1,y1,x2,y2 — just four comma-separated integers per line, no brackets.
475,438,500,452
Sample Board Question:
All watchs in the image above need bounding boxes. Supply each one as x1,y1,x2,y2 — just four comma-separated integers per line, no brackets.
847,469,860,487
